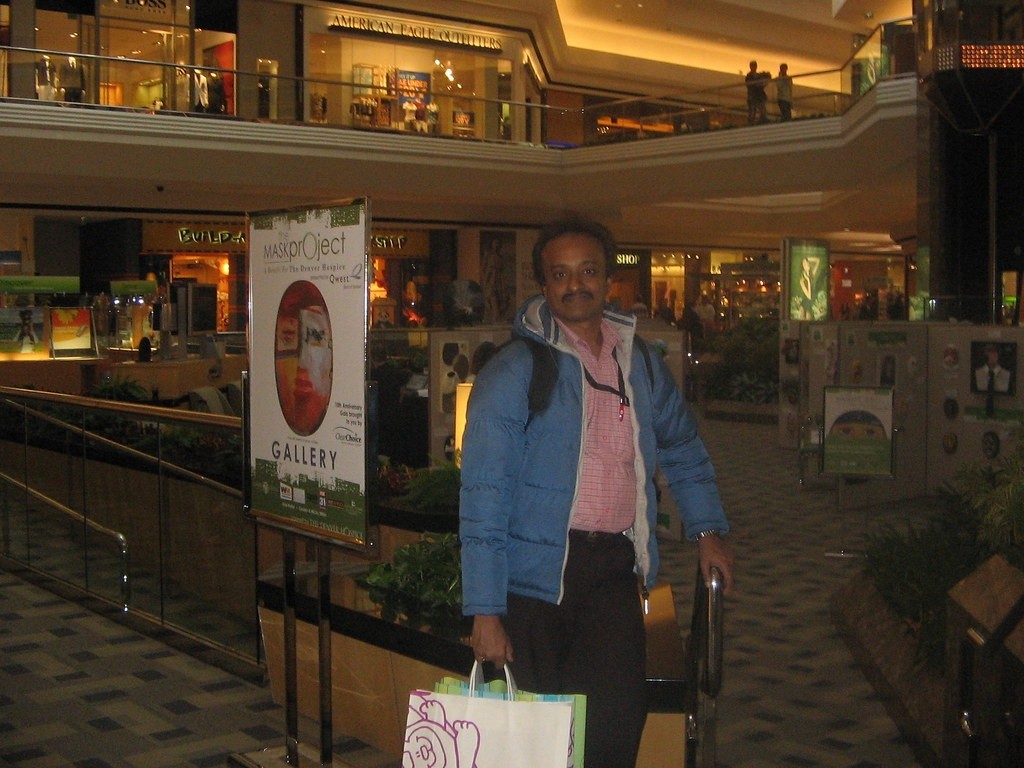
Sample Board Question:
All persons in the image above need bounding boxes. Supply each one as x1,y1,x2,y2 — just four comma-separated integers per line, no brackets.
746,60,794,122
37,54,86,108
460,216,735,768
654,288,717,348
974,344,1012,392
185,69,226,117
795,255,820,320
402,92,429,134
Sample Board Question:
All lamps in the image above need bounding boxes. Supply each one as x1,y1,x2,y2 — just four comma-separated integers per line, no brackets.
457,383,473,472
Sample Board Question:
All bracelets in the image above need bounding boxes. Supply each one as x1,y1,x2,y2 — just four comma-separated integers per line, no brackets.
693,529,715,540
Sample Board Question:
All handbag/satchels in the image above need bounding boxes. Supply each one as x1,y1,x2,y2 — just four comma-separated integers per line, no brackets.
400,658,586,768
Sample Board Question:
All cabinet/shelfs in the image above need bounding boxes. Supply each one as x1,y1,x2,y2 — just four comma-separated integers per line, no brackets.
354,63,400,130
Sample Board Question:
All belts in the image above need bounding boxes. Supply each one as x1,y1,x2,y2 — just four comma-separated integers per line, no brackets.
569,529,623,540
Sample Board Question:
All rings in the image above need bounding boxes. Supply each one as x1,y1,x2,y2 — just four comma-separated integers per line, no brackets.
479,656,486,662
